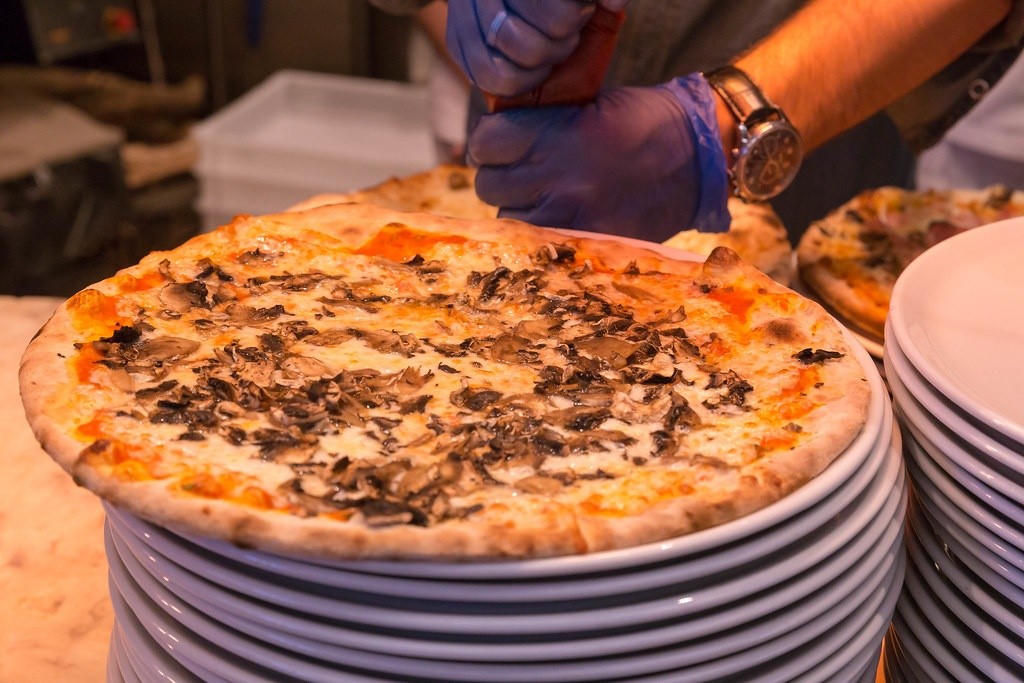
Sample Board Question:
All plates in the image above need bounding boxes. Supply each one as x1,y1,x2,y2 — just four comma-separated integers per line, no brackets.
105,216,1024,683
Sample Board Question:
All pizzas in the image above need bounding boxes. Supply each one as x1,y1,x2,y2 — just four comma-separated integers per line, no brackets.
16,203,870,560
286,162,798,293
798,182,1024,342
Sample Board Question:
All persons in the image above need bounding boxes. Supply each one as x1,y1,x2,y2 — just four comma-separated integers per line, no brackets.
446,0,1023,244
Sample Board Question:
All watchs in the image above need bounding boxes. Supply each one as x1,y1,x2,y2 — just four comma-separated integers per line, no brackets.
702,64,806,204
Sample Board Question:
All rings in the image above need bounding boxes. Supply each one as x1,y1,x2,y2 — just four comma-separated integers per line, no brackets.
486,10,508,49
